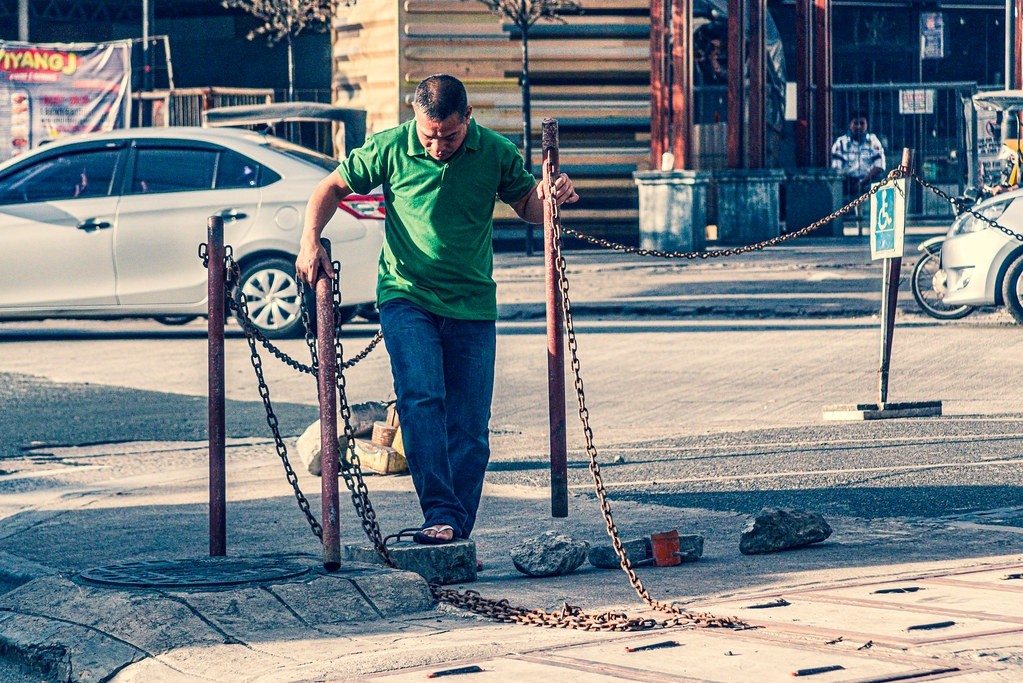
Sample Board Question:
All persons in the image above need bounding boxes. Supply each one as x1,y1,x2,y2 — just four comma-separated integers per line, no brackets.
830,113,886,197
73,162,98,199
295,74,579,571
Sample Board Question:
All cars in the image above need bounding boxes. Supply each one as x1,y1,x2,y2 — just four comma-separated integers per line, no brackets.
932,178,1023,324
0,126,385,339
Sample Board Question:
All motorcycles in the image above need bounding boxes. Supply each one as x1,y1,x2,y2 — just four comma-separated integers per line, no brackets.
973,89,1022,186
910,186,975,319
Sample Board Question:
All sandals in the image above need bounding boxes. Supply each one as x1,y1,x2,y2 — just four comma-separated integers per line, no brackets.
413,524,456,545
476,559,484,571
